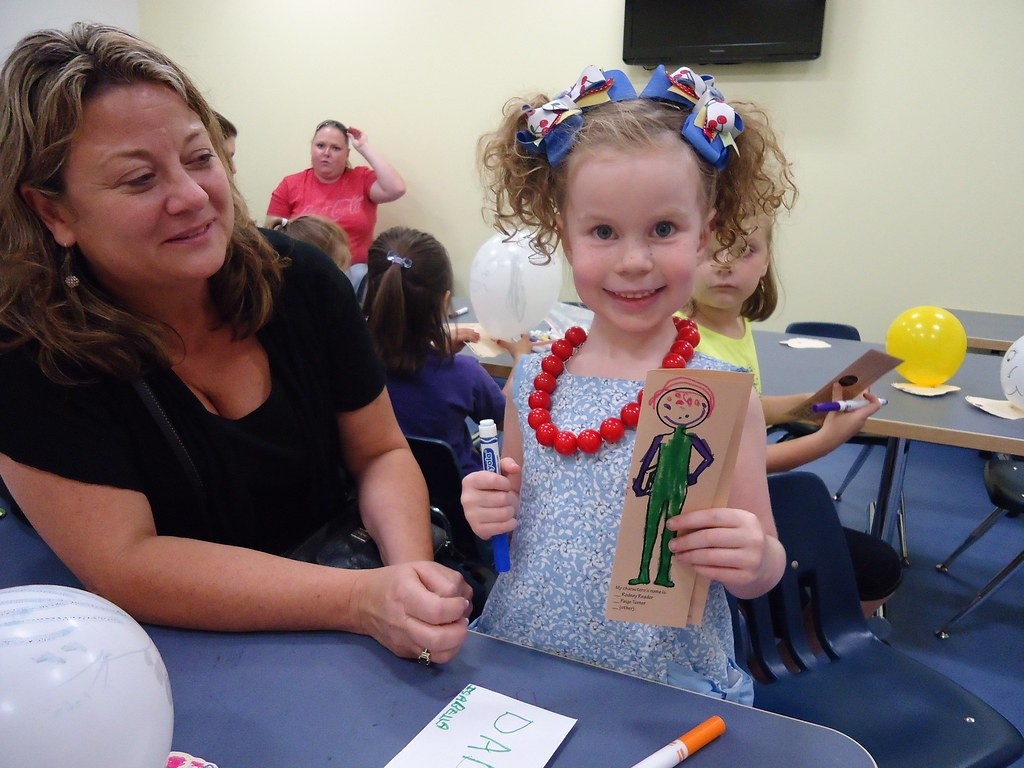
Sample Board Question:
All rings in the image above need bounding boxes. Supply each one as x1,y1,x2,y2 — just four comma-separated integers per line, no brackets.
419,649,430,666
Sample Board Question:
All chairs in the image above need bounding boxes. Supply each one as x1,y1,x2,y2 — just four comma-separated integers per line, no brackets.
934,459,1024,639
761,321,901,550
713,469,1024,768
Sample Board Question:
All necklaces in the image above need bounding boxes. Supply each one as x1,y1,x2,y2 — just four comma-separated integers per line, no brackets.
528,317,700,455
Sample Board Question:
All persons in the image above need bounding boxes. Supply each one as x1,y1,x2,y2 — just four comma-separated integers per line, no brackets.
474,64,787,712
674,165,905,687
1,21,535,663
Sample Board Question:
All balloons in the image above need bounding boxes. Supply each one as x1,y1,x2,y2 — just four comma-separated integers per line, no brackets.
468,229,564,343
1001,332,1024,410
886,305,968,390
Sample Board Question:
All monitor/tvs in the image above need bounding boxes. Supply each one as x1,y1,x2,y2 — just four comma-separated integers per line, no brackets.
623,0,825,65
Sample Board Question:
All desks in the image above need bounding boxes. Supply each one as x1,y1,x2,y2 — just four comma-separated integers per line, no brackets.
448,332,1024,563
0,494,874,768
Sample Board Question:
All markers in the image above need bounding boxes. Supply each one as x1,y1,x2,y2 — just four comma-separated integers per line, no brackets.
528,330,557,342
630,714,728,768
479,419,510,572
814,399,888,412
449,307,468,319
